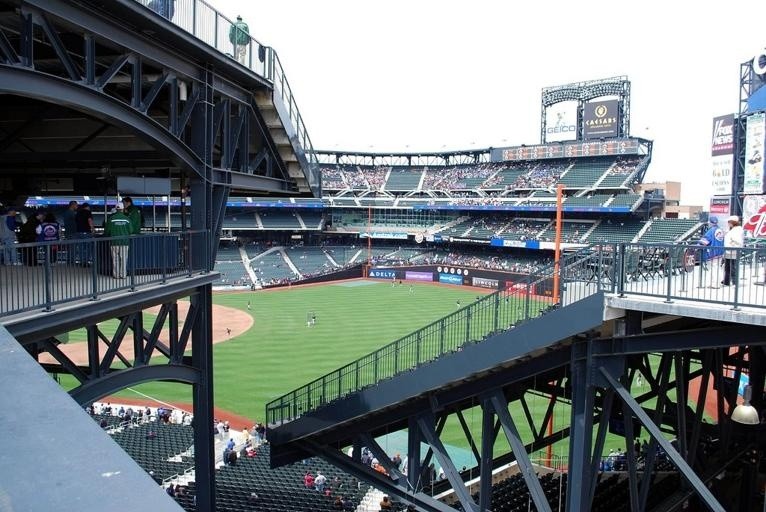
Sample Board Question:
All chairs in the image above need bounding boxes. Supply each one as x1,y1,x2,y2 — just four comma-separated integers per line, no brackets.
591,432,766,511
92,411,395,510
585,247,668,286
405,460,570,512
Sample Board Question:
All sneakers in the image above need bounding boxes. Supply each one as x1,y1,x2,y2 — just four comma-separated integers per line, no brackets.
114,275,126,279
6,262,23,266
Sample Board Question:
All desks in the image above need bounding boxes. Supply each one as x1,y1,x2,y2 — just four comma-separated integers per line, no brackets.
92,232,178,275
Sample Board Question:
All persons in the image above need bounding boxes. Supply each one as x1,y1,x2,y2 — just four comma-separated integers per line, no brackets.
247,300,251,310
637,373,642,387
457,298,461,309
228,14,250,66
301,446,468,511
609,155,641,175
305,321,313,328
422,158,573,208
84,401,266,507
721,215,745,286
599,437,668,472
696,216,725,291
408,284,415,295
312,313,315,324
475,295,480,301
456,213,624,244
319,165,390,191
225,328,231,335
217,230,660,290
0,196,142,279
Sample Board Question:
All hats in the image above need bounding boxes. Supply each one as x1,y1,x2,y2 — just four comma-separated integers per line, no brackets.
115,203,124,210
728,215,739,221
710,217,717,223
